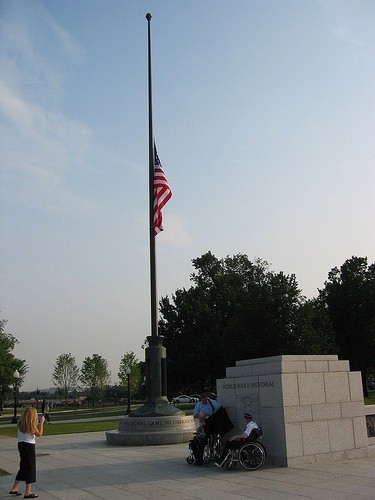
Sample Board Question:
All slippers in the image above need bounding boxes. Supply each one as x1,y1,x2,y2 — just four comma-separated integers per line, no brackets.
24,494,38,498
9,491,22,495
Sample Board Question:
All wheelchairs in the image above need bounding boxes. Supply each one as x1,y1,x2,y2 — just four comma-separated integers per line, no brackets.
186,432,225,465
213,426,267,471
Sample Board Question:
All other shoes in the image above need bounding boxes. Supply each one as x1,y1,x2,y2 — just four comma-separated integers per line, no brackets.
198,460,203,466
194,461,198,465
215,456,224,467
226,463,233,470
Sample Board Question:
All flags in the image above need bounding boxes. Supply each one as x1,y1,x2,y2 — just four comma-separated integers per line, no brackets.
152,137,172,237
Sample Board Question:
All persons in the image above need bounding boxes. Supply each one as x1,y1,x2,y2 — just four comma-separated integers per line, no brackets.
41,399,52,425
214,414,262,467
192,393,221,465
9,406,45,498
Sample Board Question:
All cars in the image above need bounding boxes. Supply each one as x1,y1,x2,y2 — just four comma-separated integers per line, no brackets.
8,403,26,408
172,391,217,403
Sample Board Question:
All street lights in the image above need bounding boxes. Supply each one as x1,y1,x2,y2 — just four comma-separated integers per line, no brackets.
125,367,132,413
10,369,20,423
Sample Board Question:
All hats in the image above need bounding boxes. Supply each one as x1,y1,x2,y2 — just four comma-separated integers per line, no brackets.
244,414,252,419
